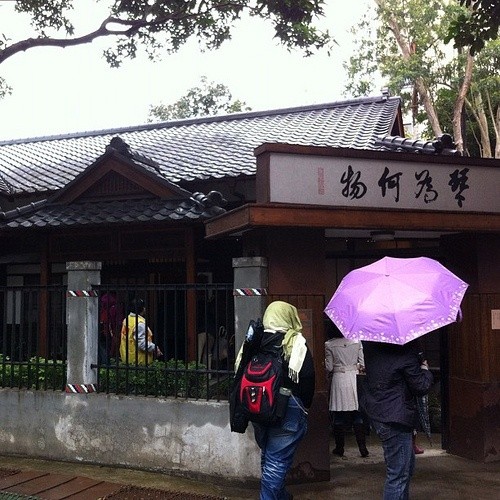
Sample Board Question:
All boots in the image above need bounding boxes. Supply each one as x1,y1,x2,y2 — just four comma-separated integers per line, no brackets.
332,434,344,457
356,439,369,457
413,439,424,454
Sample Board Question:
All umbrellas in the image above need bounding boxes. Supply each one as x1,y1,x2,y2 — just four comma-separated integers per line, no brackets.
416,353,433,442
324,256,468,346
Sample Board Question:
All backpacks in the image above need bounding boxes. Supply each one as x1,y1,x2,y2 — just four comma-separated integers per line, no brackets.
238,348,292,428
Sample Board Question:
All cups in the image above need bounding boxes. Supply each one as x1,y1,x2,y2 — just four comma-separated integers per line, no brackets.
279,387,292,396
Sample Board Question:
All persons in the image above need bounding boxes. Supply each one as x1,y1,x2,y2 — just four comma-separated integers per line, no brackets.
234,301,315,500
101,279,126,358
325,323,369,457
361,340,433,500
198,320,224,379
119,296,163,367
